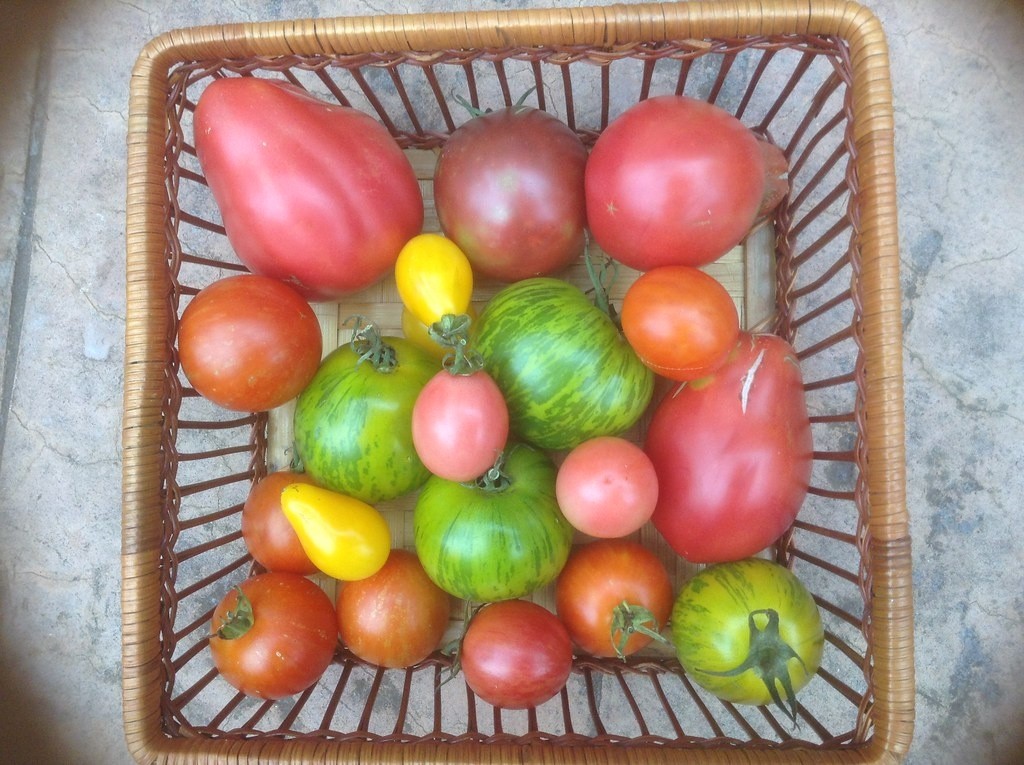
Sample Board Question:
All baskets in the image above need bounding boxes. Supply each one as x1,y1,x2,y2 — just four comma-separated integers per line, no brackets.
123,0,916,765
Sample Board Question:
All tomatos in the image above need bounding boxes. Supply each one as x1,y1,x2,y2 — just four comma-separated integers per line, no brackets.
179,76,826,709
